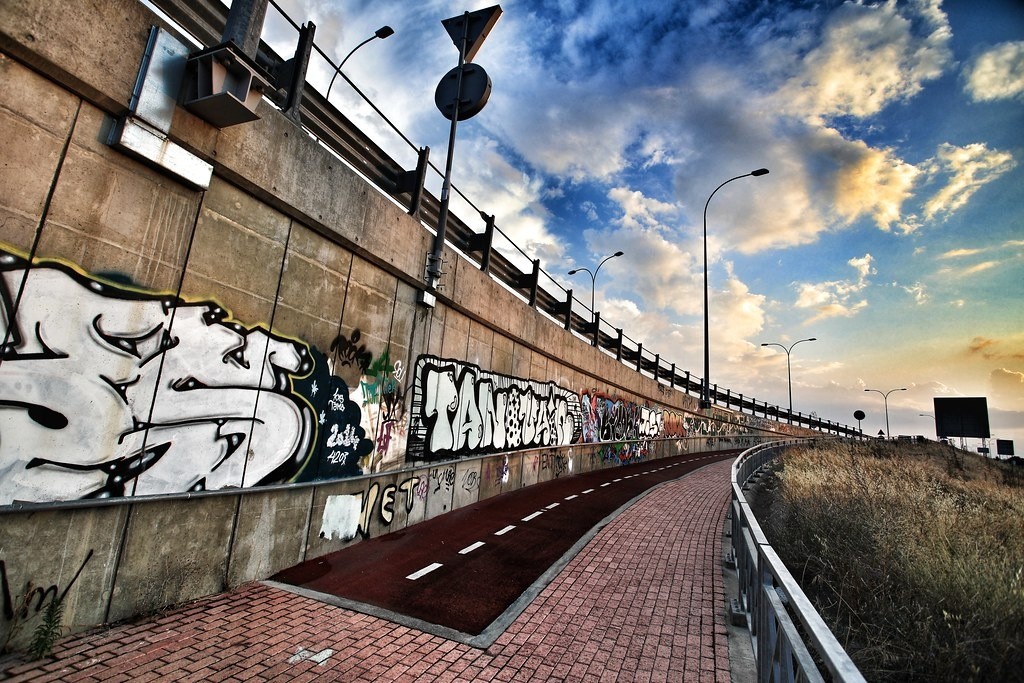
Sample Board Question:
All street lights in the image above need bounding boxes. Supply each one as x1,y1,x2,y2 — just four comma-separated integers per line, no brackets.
702,166,772,400
865,388,911,443
313,26,396,145
760,338,818,426
567,250,625,345
